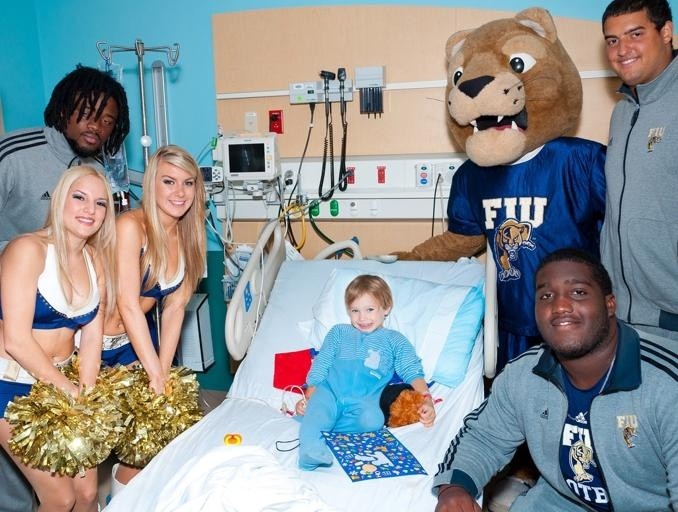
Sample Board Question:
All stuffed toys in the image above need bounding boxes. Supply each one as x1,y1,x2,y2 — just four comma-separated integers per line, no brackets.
375,379,428,429
380,4,616,512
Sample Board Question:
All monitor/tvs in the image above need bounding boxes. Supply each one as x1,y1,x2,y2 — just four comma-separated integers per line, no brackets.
222,132,282,181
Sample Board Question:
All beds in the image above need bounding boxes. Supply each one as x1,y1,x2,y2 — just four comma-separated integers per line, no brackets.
96,219,498,511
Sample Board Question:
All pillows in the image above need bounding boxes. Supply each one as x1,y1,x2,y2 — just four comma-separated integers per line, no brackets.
297,266,485,388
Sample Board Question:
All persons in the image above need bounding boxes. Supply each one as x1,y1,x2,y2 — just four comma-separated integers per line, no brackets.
580,0,678,339
292,273,438,473
1,164,119,510
0,63,132,250
428,245,678,511
69,141,211,504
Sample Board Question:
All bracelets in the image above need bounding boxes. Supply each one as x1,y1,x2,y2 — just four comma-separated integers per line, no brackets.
422,393,433,400
438,484,465,500
